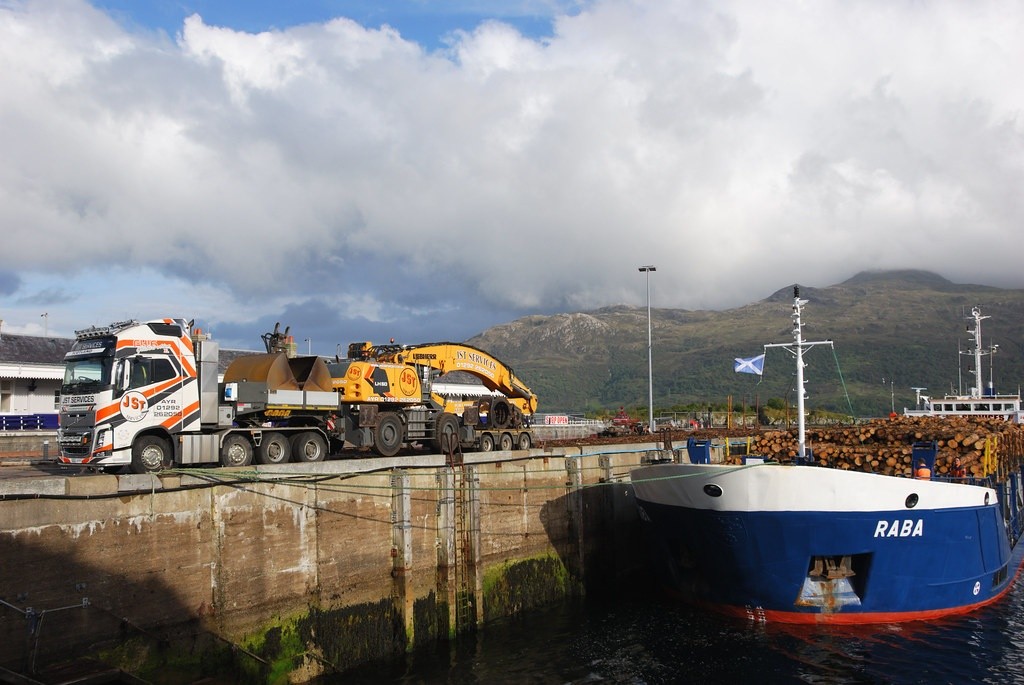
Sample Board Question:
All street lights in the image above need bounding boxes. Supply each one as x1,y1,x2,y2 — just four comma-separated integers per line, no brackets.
638,264,665,434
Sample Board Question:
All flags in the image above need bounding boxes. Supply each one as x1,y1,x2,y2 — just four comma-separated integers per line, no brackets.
734,354,764,375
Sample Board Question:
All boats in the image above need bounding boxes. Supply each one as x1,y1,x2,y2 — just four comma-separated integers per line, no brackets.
902,300,1024,427
628,283,1024,629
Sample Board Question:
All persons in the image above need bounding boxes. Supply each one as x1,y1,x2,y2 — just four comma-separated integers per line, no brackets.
912,458,931,480
949,457,966,483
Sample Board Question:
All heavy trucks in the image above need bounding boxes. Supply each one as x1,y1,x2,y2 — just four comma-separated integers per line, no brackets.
54,318,331,474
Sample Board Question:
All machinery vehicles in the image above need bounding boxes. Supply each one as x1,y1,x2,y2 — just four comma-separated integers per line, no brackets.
219,318,538,458
